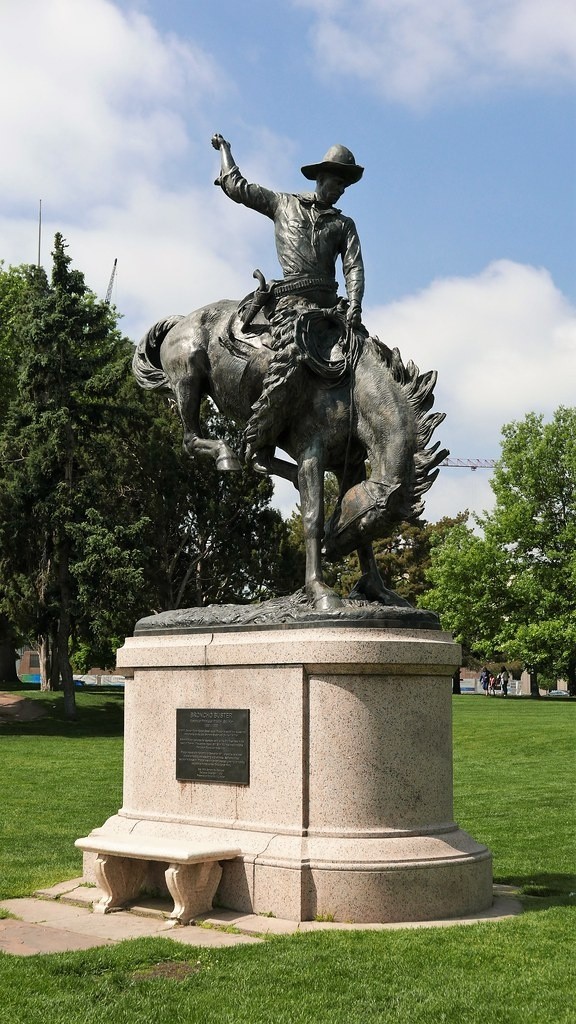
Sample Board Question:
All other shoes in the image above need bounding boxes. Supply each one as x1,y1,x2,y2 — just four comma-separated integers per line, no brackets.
504,694,506,696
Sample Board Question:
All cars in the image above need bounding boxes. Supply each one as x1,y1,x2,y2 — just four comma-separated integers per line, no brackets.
550,690,570,696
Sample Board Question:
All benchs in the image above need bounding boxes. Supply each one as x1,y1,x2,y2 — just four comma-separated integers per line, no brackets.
71,836,243,921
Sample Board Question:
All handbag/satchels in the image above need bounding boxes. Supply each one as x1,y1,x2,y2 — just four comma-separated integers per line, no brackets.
507,675,510,678
484,676,488,683
492,682,495,687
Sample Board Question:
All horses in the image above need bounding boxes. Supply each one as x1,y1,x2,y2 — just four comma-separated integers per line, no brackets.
132,298,451,610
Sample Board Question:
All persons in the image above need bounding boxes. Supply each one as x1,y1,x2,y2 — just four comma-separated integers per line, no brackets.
479,666,508,697
210,133,365,474
